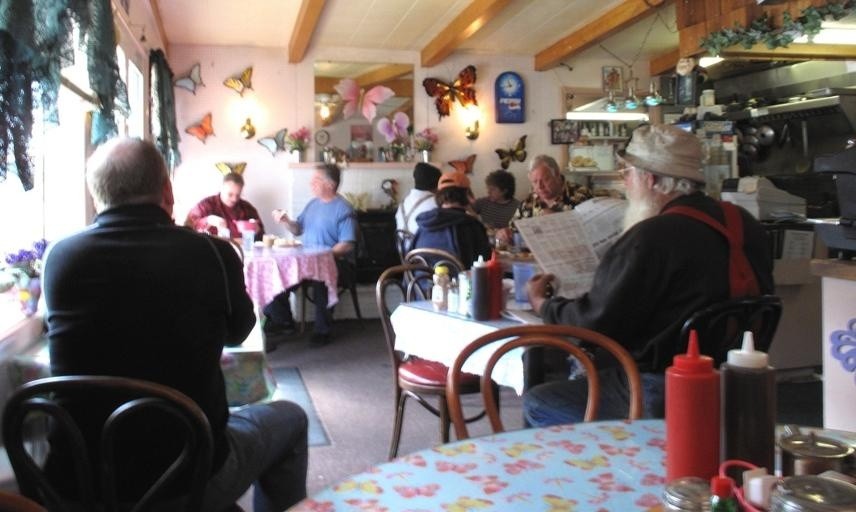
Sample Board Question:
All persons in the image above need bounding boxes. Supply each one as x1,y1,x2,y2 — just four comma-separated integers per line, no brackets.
523,122,775,431
468,170,524,238
41,136,309,511
183,172,266,241
495,155,623,249
395,160,442,266
403,171,493,303
264,163,360,347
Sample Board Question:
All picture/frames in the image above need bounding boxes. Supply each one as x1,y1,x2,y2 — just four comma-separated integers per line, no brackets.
550,118,579,144
602,66,625,93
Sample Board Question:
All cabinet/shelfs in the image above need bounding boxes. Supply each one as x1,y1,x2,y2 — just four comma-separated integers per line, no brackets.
566,135,629,176
353,208,403,284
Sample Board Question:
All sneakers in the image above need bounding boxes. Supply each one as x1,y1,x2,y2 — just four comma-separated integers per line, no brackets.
308,333,337,347
263,322,295,336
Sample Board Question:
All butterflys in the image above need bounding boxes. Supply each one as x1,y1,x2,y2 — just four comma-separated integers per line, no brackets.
492,135,530,170
221,66,255,97
422,64,480,117
256,128,289,157
172,62,206,95
447,151,480,174
333,77,396,126
213,161,247,178
183,112,217,145
375,110,411,144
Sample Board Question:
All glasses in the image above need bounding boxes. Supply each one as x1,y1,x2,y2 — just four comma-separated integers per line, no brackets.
615,166,636,180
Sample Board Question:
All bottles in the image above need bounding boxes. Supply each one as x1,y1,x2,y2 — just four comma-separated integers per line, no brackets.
430,263,449,311
485,250,501,319
661,327,719,480
718,328,776,478
578,121,627,137
469,254,489,321
701,476,741,512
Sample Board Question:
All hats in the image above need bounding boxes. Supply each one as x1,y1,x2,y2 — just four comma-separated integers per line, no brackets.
414,162,441,189
615,122,706,186
437,171,470,191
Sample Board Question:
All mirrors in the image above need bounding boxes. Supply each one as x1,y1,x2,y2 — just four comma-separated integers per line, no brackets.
311,59,416,162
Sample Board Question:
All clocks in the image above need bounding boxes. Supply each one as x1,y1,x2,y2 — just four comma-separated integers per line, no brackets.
494,70,525,123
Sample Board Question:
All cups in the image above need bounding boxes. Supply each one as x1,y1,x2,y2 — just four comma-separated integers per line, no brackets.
262,234,273,249
778,431,855,479
512,263,534,302
763,472,856,512
241,230,254,255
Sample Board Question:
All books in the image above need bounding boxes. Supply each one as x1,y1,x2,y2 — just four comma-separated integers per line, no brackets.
515,196,630,301
